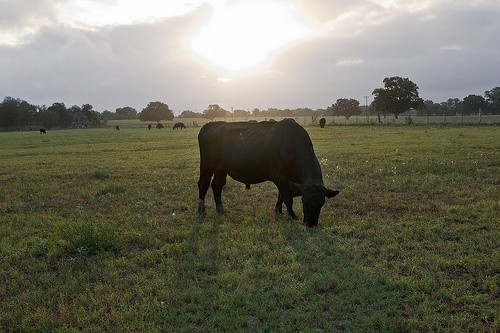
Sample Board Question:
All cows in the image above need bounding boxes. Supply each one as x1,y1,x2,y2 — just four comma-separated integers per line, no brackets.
172,122,186,130
39,128,47,135
147,124,152,130
197,118,341,227
319,118,326,128
115,126,121,131
157,123,165,129
